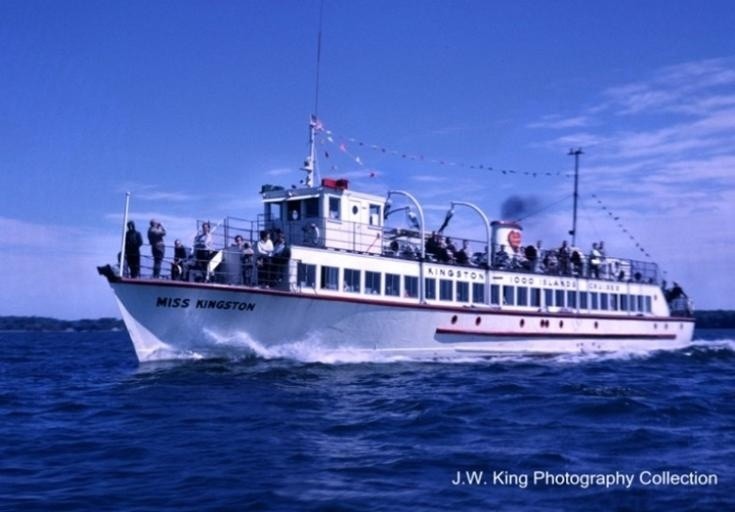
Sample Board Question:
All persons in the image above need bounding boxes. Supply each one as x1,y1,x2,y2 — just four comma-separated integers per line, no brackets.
171,210,320,289
124,221,143,279
112,252,129,278
386,231,688,303
148,219,167,279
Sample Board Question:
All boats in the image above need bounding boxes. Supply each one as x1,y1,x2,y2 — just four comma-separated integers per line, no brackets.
91,6,695,378
